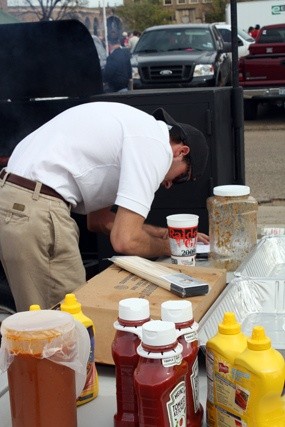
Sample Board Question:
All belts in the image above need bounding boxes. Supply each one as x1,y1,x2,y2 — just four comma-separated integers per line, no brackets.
0,169,63,199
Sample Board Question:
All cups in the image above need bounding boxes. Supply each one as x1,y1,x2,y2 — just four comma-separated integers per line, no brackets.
1,310,78,427
166,213,200,267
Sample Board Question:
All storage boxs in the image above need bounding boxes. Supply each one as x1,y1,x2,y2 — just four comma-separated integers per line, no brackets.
57,261,227,365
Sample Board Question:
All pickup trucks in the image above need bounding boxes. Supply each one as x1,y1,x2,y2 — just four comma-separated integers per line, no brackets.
239,23,285,121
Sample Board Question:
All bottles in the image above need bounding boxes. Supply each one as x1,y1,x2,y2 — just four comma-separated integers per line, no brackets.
205,312,285,427
207,184,258,271
30,293,100,407
112,297,204,427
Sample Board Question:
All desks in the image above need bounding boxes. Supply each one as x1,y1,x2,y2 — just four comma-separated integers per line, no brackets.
0,237,285,427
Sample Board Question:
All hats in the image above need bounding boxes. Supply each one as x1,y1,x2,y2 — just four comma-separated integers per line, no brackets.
154,108,209,185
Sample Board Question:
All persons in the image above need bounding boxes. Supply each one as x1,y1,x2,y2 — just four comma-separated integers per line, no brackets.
106,30,141,92
248,25,261,39
0,101,210,313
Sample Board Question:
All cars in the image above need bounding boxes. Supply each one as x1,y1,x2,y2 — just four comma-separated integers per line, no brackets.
90,20,256,89
